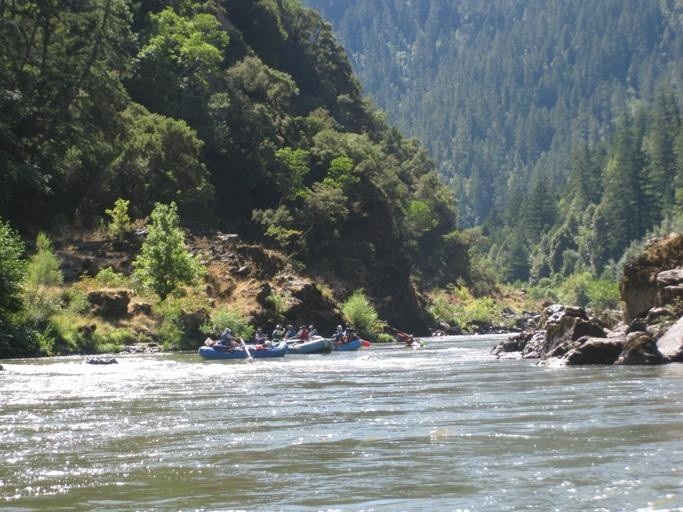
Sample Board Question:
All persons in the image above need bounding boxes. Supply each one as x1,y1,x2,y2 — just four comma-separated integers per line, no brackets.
332,321,356,346
256,324,317,348
219,328,241,347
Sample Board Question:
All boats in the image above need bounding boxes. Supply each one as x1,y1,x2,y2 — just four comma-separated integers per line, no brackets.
286,335,361,353
198,342,288,359
